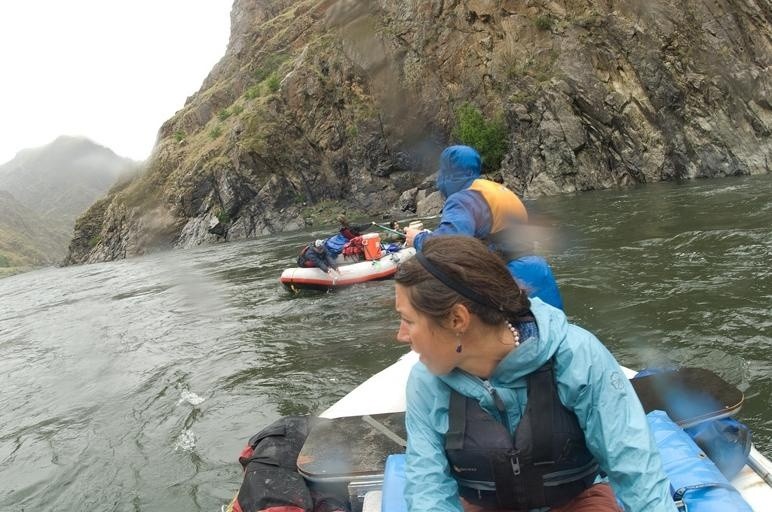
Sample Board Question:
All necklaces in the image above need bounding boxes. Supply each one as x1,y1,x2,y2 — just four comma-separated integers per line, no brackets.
503,320,521,349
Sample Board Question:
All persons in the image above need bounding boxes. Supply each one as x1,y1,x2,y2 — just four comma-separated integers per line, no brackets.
393,234,679,512
407,145,563,309
391,220,408,234
336,213,376,239
297,239,343,278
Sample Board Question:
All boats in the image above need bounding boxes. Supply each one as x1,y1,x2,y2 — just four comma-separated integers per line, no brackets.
223,340,771,512
276,240,417,298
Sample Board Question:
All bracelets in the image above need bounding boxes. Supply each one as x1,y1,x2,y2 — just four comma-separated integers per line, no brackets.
326,269,332,273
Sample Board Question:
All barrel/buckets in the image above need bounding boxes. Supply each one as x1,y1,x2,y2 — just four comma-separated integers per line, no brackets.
362,232,382,260
